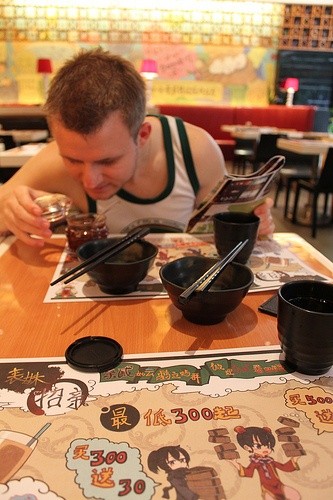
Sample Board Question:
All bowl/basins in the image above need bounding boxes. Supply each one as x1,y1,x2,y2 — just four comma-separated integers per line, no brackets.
77,237,158,295
159,255,254,325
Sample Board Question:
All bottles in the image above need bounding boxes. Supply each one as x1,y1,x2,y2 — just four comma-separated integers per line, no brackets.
66,212,110,258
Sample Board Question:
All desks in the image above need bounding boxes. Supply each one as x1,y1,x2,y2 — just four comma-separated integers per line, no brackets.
277,138,333,153
0,143,48,167
0,232,333,500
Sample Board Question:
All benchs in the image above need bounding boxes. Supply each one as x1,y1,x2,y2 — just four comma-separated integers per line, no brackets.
155,105,315,161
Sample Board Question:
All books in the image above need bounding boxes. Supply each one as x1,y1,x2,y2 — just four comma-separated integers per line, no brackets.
184,155,285,234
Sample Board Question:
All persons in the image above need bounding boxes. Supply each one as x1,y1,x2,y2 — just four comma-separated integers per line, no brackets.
0,45,276,246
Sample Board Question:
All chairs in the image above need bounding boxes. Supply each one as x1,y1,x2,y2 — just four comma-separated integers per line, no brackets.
232,134,288,172
291,147,333,239
274,153,313,219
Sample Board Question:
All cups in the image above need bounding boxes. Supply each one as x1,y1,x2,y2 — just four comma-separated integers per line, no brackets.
213,212,260,264
277,280,333,375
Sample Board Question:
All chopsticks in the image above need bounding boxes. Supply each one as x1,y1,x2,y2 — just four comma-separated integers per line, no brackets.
180,239,249,300
50,227,150,286
